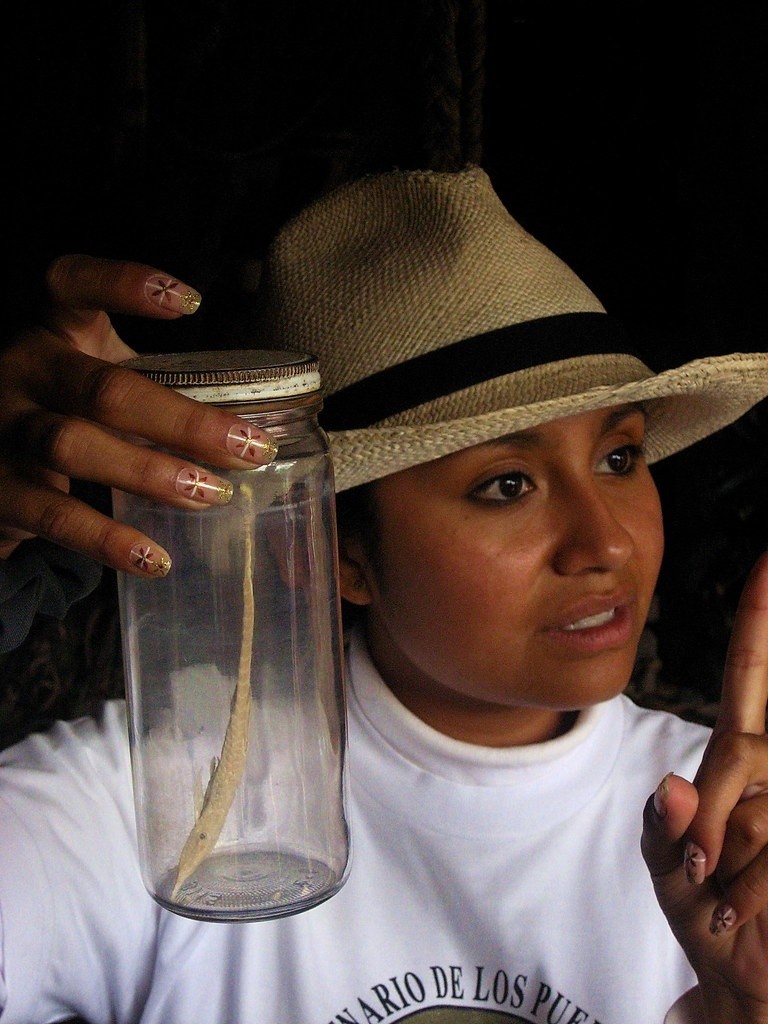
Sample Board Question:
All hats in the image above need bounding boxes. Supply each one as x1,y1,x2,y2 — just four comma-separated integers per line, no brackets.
114,162,768,514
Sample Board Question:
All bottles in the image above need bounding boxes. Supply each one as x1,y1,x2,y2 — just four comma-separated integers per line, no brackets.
113,349,355,925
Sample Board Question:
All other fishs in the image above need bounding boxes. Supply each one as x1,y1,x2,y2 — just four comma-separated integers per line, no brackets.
166,481,260,900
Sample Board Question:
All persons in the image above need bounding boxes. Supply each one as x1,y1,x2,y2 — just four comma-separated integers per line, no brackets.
0,164,768,1024
